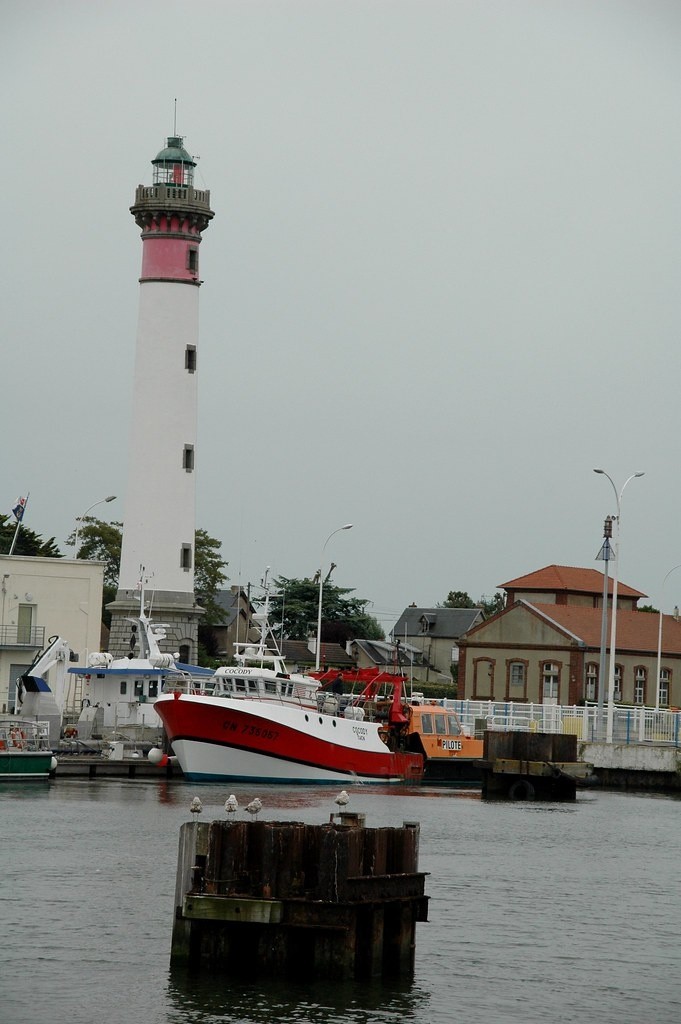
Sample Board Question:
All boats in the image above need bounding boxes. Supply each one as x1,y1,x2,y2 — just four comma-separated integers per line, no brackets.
152,565,424,781
377,638,484,780
0,720,53,776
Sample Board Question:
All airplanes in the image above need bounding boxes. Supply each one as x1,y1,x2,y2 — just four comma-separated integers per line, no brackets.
16,563,323,773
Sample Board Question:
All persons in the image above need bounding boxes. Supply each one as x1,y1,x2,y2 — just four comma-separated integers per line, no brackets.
323,694,338,713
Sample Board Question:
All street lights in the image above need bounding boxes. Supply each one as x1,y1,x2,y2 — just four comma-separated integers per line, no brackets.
593,468,646,744
75,495,117,559
316,524,354,674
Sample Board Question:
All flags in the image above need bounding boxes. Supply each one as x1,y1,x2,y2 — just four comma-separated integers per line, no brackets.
12,497,25,520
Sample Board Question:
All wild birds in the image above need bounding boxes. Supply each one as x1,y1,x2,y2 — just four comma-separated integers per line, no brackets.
334,790,350,812
224,794,238,821
189,797,203,822
244,798,262,821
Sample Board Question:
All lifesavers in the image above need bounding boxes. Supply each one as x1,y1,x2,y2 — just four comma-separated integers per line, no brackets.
481,783,488,800
508,780,535,802
12,728,26,748
